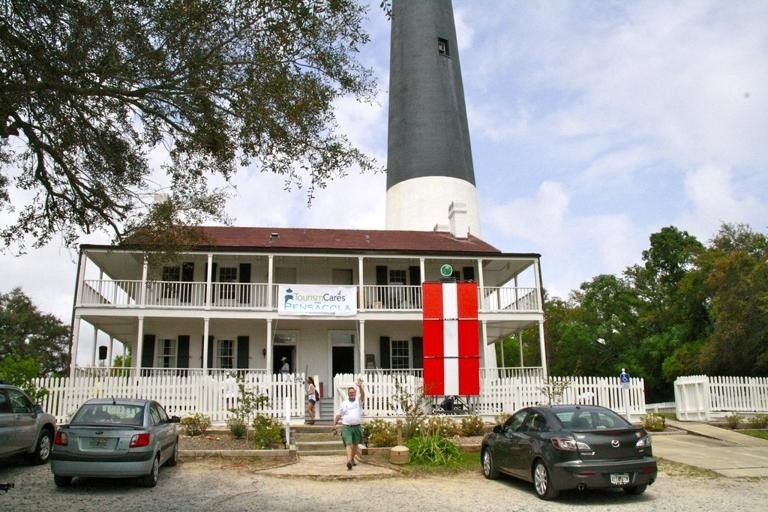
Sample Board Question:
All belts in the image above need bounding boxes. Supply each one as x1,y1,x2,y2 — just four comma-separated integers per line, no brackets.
346,424,361,427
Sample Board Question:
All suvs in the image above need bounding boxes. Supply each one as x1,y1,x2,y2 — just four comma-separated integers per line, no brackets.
0,382,59,467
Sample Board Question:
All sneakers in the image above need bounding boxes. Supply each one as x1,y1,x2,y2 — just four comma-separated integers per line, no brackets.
351,458,357,466
346,460,353,469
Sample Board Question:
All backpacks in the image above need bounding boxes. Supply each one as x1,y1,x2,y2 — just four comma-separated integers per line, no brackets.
315,389,320,402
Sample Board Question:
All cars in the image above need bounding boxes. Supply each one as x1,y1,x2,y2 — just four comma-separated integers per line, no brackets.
44,392,184,487
476,399,659,503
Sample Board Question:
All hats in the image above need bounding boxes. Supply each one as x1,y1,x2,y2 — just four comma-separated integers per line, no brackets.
281,356,287,361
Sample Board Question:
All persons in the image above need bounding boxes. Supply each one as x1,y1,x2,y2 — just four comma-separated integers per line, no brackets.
278,356,290,373
330,376,366,470
302,376,320,425
